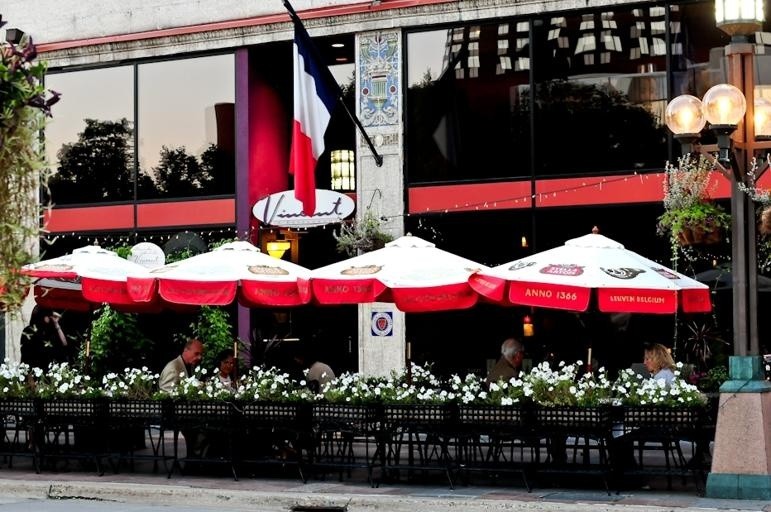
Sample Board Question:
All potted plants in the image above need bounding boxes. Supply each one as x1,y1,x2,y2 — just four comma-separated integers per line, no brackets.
738,154,771,273
657,153,732,266
332,208,393,257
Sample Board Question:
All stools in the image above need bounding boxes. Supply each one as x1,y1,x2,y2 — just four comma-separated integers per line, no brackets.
2,421,693,480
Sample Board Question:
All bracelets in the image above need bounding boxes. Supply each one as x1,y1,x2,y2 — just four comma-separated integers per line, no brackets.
56,325,61,330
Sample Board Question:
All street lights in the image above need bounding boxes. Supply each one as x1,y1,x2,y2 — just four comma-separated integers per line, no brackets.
664,0,771,502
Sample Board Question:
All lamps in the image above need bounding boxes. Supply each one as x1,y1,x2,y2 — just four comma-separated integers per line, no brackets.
266,242,290,260
330,149,357,193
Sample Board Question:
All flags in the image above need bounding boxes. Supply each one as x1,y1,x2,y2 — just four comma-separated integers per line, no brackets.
284,19,340,218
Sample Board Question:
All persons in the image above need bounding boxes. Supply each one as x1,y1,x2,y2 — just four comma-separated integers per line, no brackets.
604,343,676,470
252,353,336,477
205,349,238,391
157,339,228,478
485,337,569,464
17,306,70,451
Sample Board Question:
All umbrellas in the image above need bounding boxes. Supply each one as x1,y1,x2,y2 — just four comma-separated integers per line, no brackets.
297,231,490,386
0,243,194,371
468,225,713,375
126,240,310,387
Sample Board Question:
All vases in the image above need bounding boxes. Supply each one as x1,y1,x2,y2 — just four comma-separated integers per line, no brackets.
0,400,702,438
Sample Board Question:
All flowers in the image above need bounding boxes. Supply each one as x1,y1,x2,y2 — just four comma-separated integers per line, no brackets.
0,361,708,408
